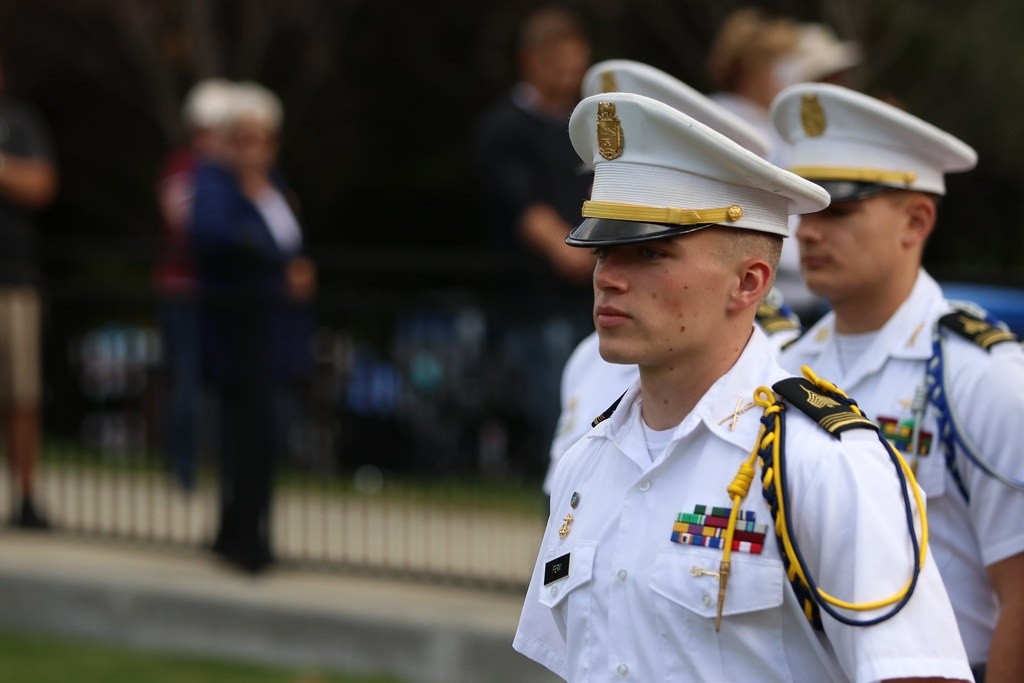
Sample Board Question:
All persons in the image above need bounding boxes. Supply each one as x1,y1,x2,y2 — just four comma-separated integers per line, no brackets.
191,90,316,577
510,92,975,683
471,8,598,522
710,9,865,349
771,82,1024,683
543,60,772,496
0,96,59,533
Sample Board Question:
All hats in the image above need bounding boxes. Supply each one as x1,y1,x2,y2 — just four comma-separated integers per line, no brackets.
769,82,978,196
566,59,831,246
